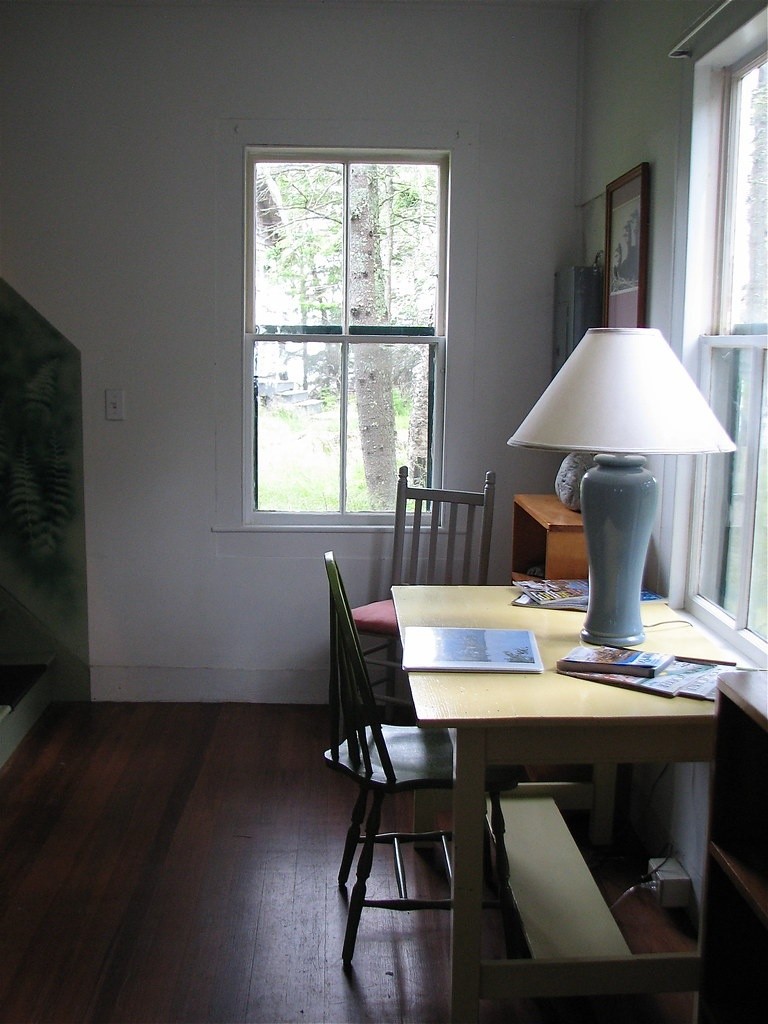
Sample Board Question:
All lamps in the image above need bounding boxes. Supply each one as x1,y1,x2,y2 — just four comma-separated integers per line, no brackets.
506,327,737,647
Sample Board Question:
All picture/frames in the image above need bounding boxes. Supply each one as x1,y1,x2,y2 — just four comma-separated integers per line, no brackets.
601,162,649,330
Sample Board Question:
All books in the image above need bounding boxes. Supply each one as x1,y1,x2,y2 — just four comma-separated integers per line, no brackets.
401,627,544,674
512,578,665,614
555,645,768,702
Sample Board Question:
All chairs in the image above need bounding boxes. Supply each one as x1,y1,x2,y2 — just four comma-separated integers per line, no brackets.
338,464,496,739
322,550,532,975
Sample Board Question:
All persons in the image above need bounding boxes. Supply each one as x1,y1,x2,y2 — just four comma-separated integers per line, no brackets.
609,209,640,291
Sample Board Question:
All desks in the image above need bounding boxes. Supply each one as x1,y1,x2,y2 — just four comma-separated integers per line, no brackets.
386,583,731,1024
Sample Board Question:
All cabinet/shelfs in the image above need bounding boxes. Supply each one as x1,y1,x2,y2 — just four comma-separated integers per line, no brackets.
696,670,768,1024
509,491,590,582
553,265,601,382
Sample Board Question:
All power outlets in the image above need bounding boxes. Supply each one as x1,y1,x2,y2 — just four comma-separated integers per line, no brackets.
646,857,691,910
